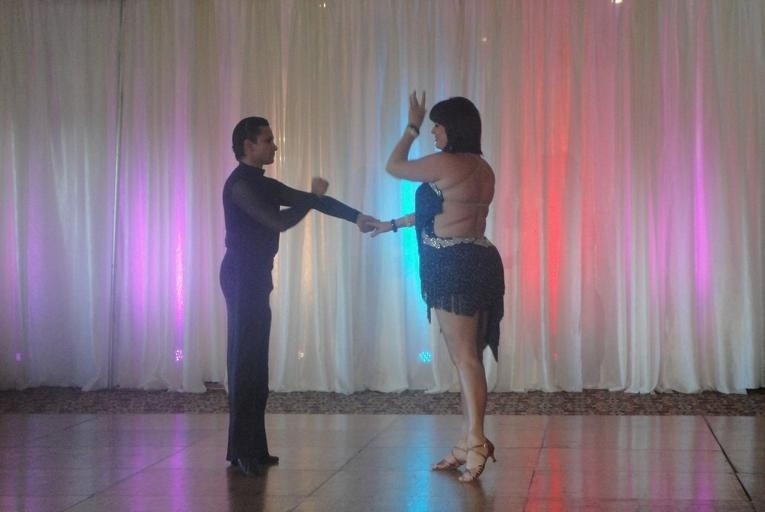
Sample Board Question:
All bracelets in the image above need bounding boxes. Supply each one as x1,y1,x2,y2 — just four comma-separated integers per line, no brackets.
391,220,397,232
407,125,419,136
405,214,411,227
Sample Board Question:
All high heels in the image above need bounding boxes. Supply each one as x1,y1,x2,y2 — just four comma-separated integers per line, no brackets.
433,447,468,471
458,436,496,482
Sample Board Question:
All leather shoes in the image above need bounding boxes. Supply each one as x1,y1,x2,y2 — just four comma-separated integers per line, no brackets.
230,451,279,476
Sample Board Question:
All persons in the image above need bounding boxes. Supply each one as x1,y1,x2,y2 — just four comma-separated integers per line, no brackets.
368,91,505,484
220,117,382,479
